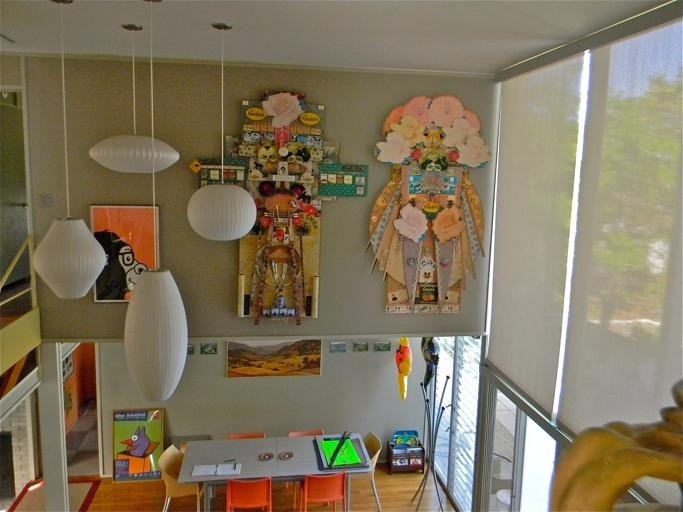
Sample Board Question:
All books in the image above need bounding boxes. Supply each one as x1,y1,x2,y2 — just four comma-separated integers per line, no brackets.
191,462,241,476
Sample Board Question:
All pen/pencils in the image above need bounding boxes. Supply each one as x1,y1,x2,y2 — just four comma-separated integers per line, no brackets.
224,459,238,470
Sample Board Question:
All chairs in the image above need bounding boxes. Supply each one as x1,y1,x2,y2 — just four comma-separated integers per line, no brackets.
157,427,384,512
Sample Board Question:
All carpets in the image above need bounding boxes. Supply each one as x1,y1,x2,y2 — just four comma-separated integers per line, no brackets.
7,480,101,512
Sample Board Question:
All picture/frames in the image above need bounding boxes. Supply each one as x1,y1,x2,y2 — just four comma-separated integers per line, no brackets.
225,340,323,378
111,408,165,483
90,203,160,304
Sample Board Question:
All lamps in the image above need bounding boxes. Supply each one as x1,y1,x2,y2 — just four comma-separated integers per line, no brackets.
187,24,258,241
32,0,107,299
122,0,191,403
89,26,183,175
